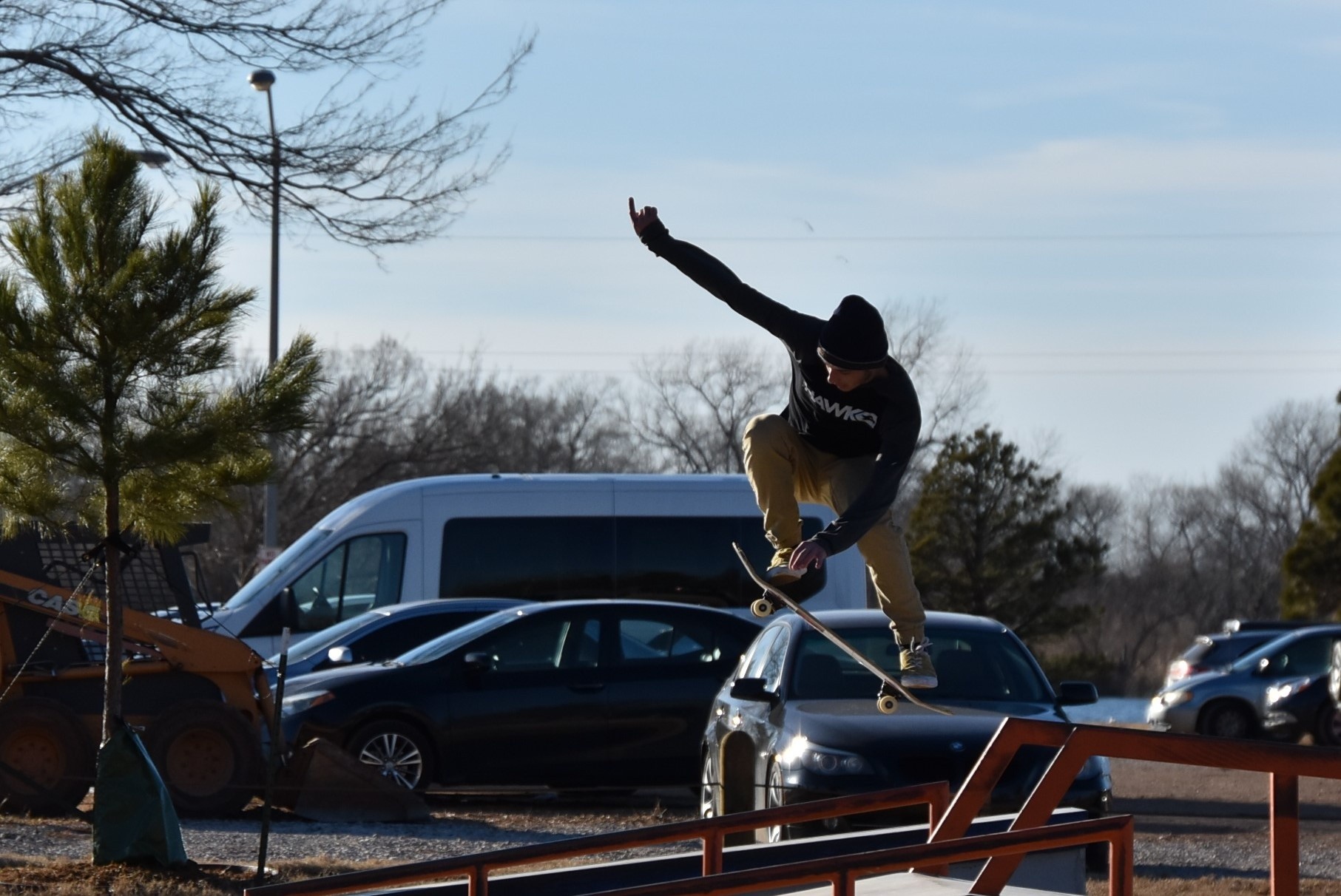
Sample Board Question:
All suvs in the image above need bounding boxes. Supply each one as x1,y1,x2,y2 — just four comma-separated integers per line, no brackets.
1168,619,1304,685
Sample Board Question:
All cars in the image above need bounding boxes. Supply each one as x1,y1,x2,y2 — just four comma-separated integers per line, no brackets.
264,595,860,806
700,611,1111,872
84,594,375,643
1147,628,1340,748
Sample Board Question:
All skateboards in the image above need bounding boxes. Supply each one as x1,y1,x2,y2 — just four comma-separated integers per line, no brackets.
731,540,956,716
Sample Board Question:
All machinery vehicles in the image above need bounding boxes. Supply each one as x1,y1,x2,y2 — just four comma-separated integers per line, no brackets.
2,519,285,818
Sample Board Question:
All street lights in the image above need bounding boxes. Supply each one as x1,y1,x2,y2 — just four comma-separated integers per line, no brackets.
247,70,282,578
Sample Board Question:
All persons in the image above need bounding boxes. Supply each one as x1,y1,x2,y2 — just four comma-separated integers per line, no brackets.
628,196,938,688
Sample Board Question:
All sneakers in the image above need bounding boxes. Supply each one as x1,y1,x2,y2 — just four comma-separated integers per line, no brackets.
763,563,806,584
899,636,938,689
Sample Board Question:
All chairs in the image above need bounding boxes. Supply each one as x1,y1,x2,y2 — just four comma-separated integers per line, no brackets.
934,649,988,700
798,654,847,699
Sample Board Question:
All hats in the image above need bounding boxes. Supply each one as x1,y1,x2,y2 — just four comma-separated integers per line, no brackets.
817,295,888,368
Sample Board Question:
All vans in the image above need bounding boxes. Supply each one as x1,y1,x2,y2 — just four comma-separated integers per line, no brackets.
142,472,870,663
